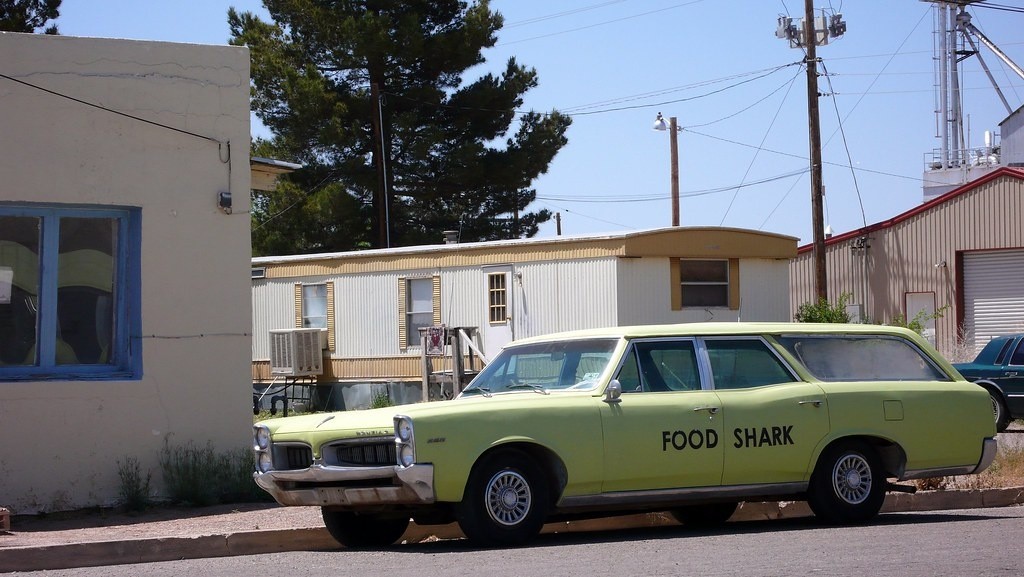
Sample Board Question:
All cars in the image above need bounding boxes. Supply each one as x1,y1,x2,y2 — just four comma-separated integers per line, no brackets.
251,318,998,549
925,332,1024,434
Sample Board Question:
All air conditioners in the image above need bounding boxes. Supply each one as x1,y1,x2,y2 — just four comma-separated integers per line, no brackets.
269,328,323,376
